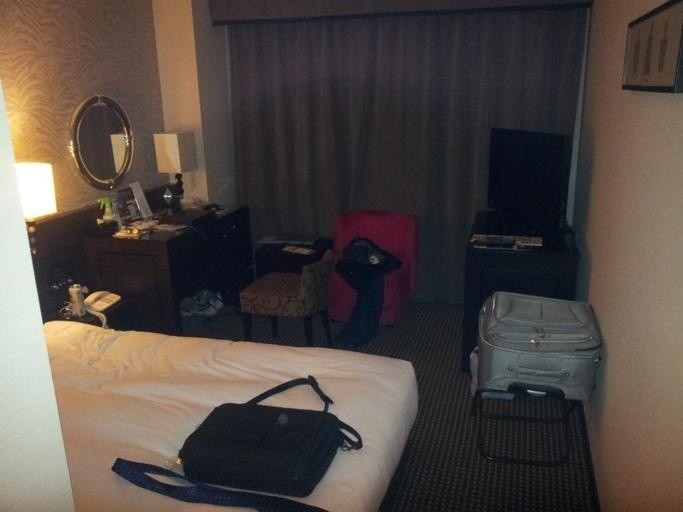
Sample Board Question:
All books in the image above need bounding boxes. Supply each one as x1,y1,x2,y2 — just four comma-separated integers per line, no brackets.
469,232,543,248
255,234,322,245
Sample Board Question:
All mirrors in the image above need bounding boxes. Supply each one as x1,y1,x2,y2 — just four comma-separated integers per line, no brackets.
64,92,135,195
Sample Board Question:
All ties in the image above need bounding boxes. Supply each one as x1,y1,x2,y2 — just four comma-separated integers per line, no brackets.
112,456,331,512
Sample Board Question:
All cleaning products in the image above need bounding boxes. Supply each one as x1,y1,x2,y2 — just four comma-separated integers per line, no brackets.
96,195,117,221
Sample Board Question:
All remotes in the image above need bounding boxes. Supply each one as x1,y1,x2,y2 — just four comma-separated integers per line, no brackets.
476,239,513,247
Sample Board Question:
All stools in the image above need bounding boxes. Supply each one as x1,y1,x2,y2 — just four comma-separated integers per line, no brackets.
464,345,583,468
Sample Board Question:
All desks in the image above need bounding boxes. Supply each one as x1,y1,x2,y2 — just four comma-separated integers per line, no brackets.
75,199,258,336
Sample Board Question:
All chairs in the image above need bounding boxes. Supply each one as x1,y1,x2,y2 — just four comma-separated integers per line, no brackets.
233,247,339,351
325,208,422,330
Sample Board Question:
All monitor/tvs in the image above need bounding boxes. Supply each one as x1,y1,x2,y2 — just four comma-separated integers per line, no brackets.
488,128,571,229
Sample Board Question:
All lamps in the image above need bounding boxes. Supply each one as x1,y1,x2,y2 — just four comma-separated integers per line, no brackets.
12,158,56,259
146,129,199,207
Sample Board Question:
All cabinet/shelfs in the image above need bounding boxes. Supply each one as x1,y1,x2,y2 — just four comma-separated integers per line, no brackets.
458,208,582,377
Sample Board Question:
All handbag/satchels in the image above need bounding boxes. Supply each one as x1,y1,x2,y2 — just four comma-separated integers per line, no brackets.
181,403,341,497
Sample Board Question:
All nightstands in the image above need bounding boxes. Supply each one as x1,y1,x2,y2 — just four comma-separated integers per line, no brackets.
54,294,130,331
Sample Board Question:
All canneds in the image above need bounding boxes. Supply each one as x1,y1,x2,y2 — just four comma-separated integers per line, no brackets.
68,283,87,317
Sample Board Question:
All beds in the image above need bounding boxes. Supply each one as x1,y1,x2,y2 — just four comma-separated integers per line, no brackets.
43,316,424,510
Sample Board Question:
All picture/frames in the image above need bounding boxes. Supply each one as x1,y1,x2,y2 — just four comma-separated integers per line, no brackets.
615,0,681,99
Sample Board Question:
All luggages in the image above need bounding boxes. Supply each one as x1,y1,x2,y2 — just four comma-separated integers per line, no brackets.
476,286,602,403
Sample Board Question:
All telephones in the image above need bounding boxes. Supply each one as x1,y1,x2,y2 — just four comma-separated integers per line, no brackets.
84,291,122,312
